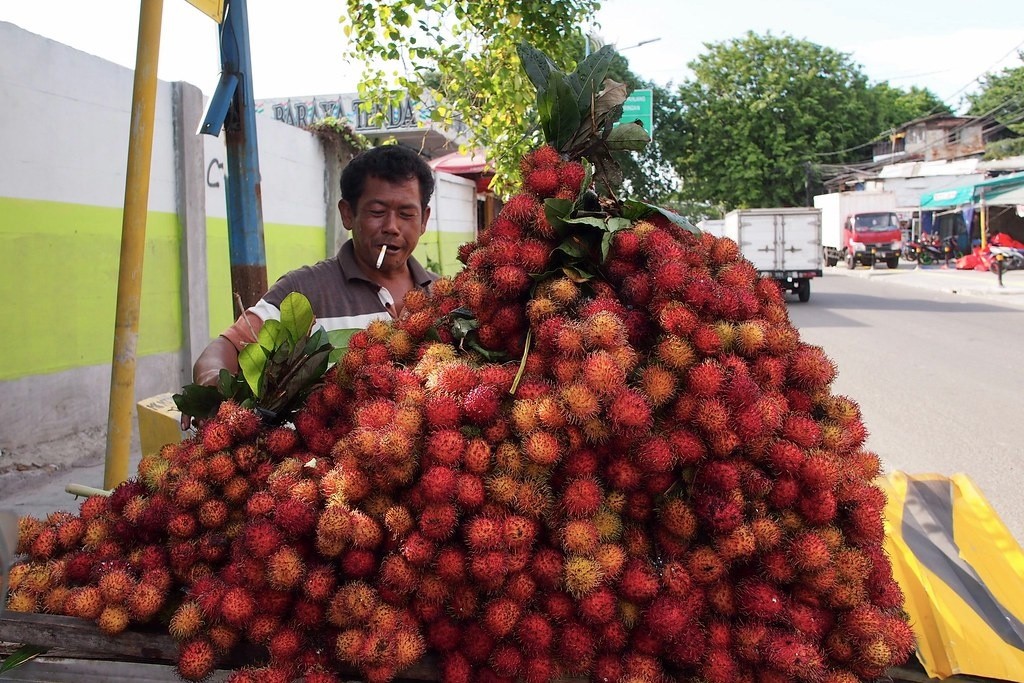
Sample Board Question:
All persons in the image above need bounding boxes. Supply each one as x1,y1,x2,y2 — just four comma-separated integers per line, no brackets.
906,230,940,246
180,144,449,433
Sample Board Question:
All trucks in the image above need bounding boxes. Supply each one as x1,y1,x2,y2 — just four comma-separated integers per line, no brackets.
725,207,823,302
813,191,902,270
696,220,727,239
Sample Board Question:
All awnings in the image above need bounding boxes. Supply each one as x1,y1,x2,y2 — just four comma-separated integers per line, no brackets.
919,167,1024,216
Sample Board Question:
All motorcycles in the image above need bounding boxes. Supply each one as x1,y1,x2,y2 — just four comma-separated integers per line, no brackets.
904,232,963,267
990,245,1024,274
